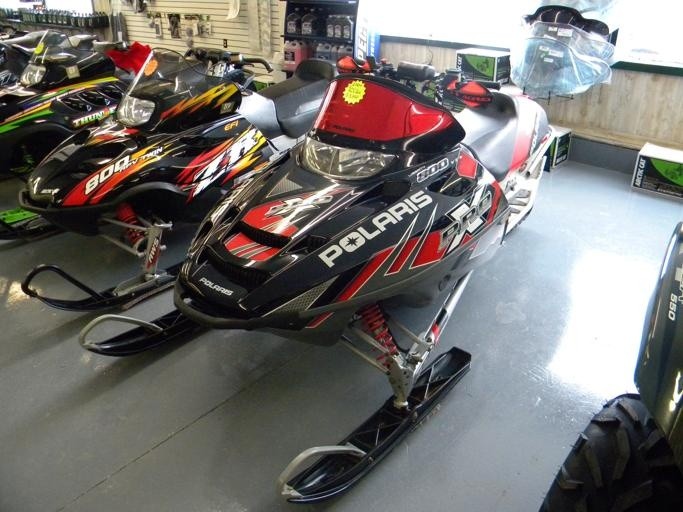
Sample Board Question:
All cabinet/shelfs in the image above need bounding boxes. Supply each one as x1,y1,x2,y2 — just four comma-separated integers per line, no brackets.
279,0,381,80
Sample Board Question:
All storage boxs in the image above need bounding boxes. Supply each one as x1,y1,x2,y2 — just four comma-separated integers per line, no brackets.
455,46,510,90
630,142,682,204
542,122,571,174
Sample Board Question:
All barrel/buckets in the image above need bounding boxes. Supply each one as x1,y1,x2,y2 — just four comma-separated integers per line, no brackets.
283,7,354,73
